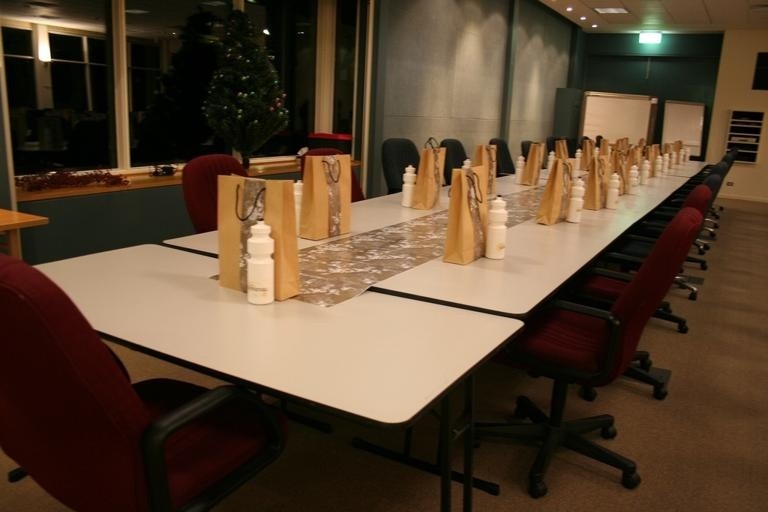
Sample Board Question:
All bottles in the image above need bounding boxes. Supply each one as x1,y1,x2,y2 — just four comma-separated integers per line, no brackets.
514,145,613,186
604,170,620,211
640,148,690,185
399,164,417,207
627,165,639,195
461,159,472,169
245,218,276,305
292,178,303,238
485,194,507,261
565,177,587,223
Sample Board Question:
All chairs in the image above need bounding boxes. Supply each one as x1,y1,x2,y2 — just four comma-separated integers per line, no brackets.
181,134,738,504
1,253,290,511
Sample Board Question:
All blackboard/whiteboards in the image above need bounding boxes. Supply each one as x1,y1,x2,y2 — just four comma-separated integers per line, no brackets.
582,91,651,149
661,100,706,160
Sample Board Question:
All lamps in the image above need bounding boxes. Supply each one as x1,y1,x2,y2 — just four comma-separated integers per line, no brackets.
636,34,662,45
36,23,53,70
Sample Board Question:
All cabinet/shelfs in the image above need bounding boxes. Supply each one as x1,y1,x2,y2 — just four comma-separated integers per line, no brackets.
724,110,765,165
552,86,582,139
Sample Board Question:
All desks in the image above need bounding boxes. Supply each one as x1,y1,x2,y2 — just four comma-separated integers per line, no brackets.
28,242,526,512
0,206,51,262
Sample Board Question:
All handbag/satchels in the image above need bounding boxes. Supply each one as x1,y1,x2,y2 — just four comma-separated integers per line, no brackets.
217,171,301,300
410,138,497,209
444,165,491,265
298,154,352,242
522,137,682,226
